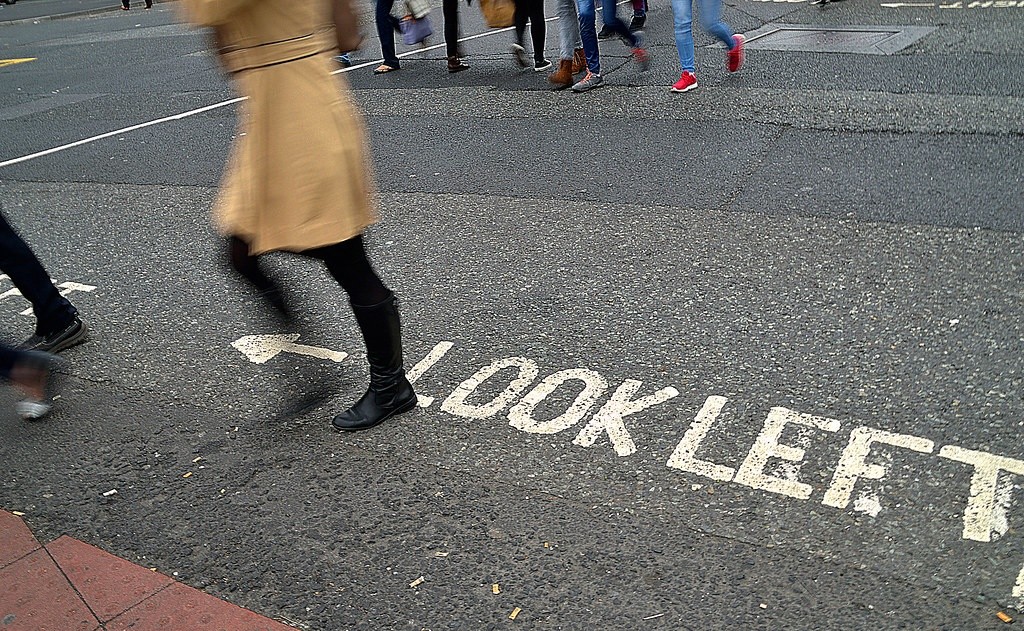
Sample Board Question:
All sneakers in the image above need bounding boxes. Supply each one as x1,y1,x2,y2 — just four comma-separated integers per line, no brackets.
669,69,700,93
728,34,747,73
571,72,605,92
633,31,651,70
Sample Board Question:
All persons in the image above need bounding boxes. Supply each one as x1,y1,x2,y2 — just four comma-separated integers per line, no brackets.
598,0,646,39
510,0,552,72
547,0,586,86
0,211,88,420
121,0,152,10
374,0,469,74
181,0,417,431
671,0,746,93
572,0,649,92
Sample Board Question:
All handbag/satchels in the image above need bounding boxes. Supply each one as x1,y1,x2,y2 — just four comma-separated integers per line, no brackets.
406,0,433,19
480,0,515,27
397,14,433,46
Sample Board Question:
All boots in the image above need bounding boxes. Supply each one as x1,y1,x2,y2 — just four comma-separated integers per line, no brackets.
573,49,588,76
327,293,418,429
549,59,576,85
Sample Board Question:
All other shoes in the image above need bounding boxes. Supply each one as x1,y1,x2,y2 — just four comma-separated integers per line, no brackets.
447,57,470,74
15,317,89,353
598,24,614,39
628,11,648,30
374,63,397,74
535,59,553,73
